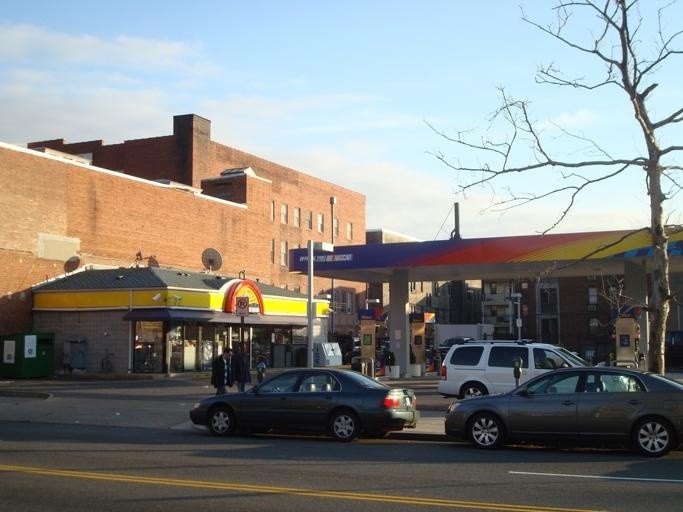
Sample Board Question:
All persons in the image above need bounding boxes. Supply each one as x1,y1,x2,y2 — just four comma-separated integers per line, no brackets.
210,348,231,395
230,345,252,391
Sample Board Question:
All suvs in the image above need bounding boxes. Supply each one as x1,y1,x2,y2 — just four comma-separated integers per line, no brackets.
437,339,593,398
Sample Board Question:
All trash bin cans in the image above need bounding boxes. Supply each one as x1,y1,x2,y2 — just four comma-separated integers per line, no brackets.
433,355,441,372
354,357,374,380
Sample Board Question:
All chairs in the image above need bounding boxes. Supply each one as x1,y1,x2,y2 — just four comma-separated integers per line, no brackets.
305,379,335,396
585,377,609,395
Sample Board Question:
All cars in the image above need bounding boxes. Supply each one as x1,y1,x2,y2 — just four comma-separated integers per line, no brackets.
189,367,418,442
443,367,683,457
